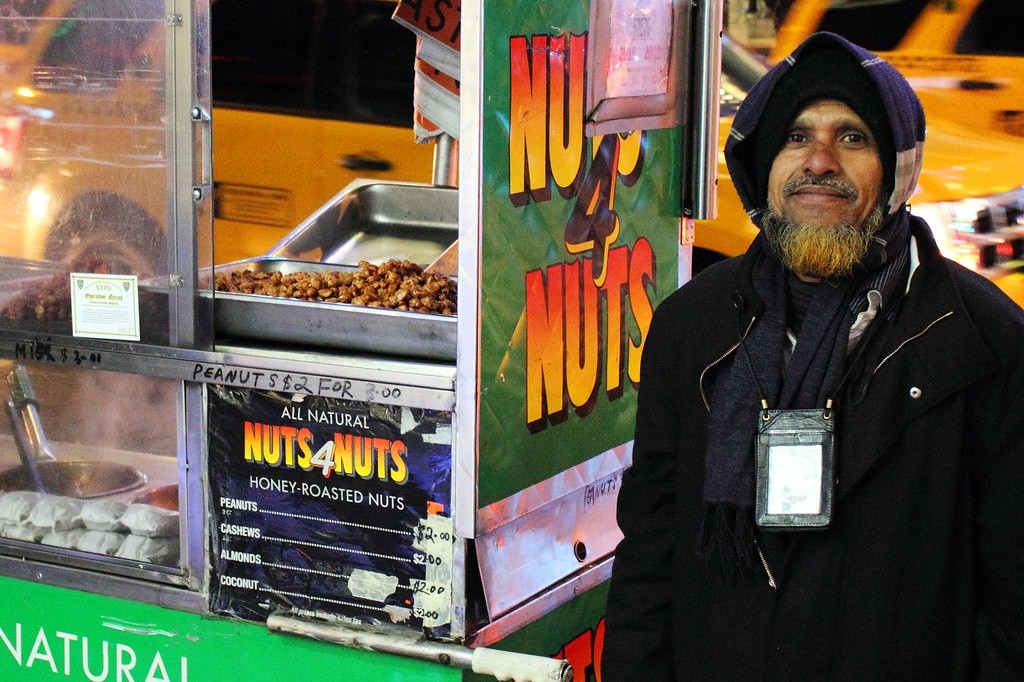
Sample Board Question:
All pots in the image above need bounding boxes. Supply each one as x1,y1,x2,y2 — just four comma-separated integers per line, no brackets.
0,365,147,499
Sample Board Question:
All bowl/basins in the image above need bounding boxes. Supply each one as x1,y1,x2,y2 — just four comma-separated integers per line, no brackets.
128,484,178,512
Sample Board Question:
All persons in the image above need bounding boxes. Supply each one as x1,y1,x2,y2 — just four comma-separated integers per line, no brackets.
601,31,1024,682
